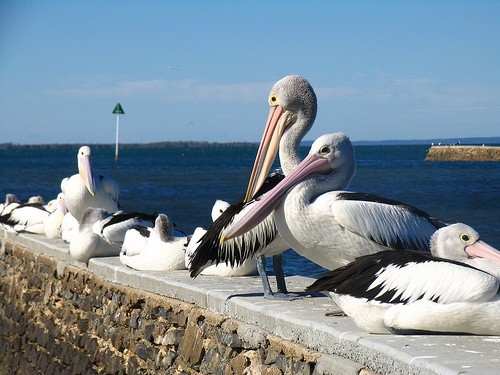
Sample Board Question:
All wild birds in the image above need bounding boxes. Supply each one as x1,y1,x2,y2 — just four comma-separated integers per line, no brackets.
0,75,500,337
